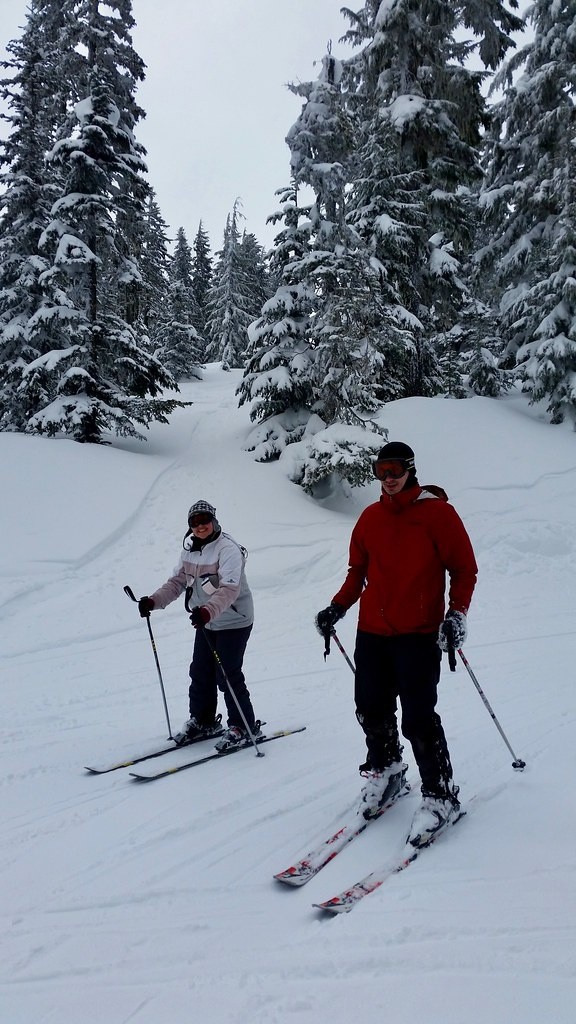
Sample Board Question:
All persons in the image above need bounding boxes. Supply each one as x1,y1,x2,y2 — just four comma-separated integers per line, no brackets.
314,442,479,845
138,499,255,749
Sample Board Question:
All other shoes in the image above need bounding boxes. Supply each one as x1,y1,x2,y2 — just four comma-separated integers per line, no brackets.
412,785,461,839
221,724,260,746
182,718,218,735
355,763,409,818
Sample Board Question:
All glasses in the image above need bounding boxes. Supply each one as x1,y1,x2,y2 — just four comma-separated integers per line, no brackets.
188,512,212,528
372,458,408,481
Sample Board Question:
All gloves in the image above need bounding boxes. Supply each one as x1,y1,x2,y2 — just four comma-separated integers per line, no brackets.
315,600,342,637
189,605,211,630
436,609,467,651
138,597,155,618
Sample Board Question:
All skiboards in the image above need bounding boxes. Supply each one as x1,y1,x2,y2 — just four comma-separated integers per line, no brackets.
83,718,308,783
272,778,510,916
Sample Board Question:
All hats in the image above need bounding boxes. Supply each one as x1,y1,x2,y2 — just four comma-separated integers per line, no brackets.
182,500,220,552
378,441,416,479
